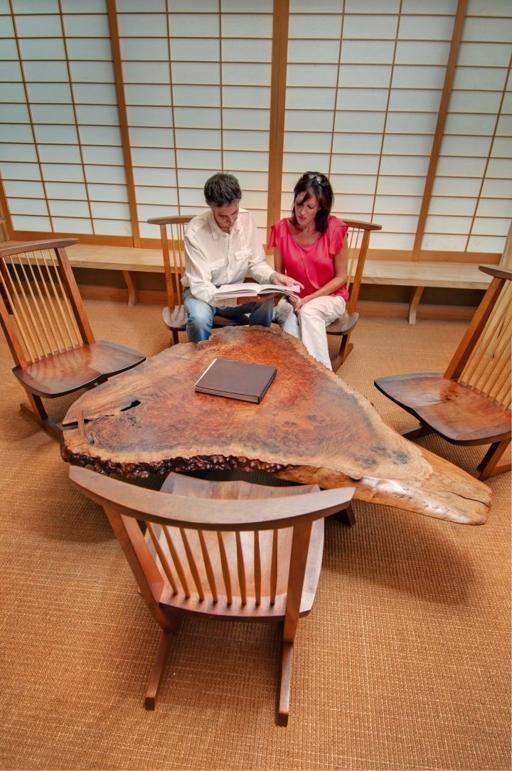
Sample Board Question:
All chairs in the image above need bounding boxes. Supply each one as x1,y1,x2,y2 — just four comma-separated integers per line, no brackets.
272,215,383,374
372,263,511,482
67,463,358,726
1,238,147,440
147,216,251,347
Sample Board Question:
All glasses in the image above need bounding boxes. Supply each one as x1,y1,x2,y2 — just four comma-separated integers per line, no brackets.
302,173,326,184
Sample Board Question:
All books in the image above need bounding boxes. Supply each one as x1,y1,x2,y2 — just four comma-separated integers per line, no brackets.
193,356,278,405
213,284,299,301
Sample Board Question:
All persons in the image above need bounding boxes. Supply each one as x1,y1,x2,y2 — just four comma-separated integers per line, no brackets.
179,172,305,343
267,172,350,371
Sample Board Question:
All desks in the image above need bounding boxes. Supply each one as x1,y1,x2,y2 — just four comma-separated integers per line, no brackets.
59,321,494,530
